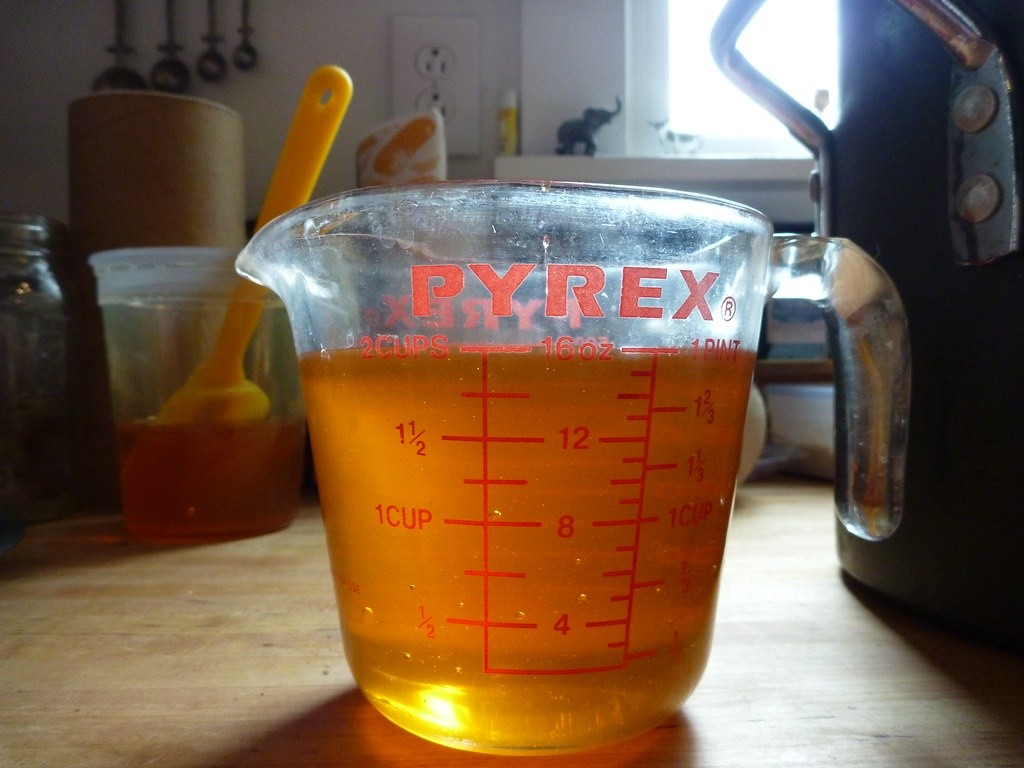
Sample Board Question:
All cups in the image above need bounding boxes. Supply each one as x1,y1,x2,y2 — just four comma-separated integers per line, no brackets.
86,245,305,545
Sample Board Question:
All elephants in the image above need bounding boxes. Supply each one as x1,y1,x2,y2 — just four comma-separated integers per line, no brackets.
555,95,621,156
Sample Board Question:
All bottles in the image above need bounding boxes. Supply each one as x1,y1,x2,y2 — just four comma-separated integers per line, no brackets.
0,215,74,529
496,89,520,156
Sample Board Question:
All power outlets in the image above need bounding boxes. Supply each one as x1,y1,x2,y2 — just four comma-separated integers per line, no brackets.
389,12,481,156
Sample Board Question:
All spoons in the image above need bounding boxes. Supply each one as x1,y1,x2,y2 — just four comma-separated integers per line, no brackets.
154,63,354,424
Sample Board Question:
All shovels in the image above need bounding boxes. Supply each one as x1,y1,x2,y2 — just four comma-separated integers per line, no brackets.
149,62,356,423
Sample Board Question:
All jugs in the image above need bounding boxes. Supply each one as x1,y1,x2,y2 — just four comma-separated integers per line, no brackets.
231,177,915,759
